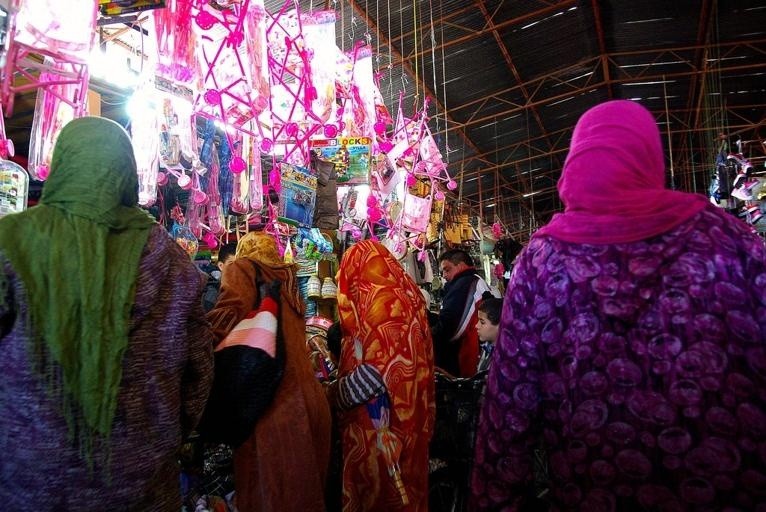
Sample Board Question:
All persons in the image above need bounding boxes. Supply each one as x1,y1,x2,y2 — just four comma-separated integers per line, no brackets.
318,240,439,512
430,297,503,511
202,230,332,512
200,245,241,313
325,318,341,369
0,115,216,512
429,248,492,377
470,99,766,511
